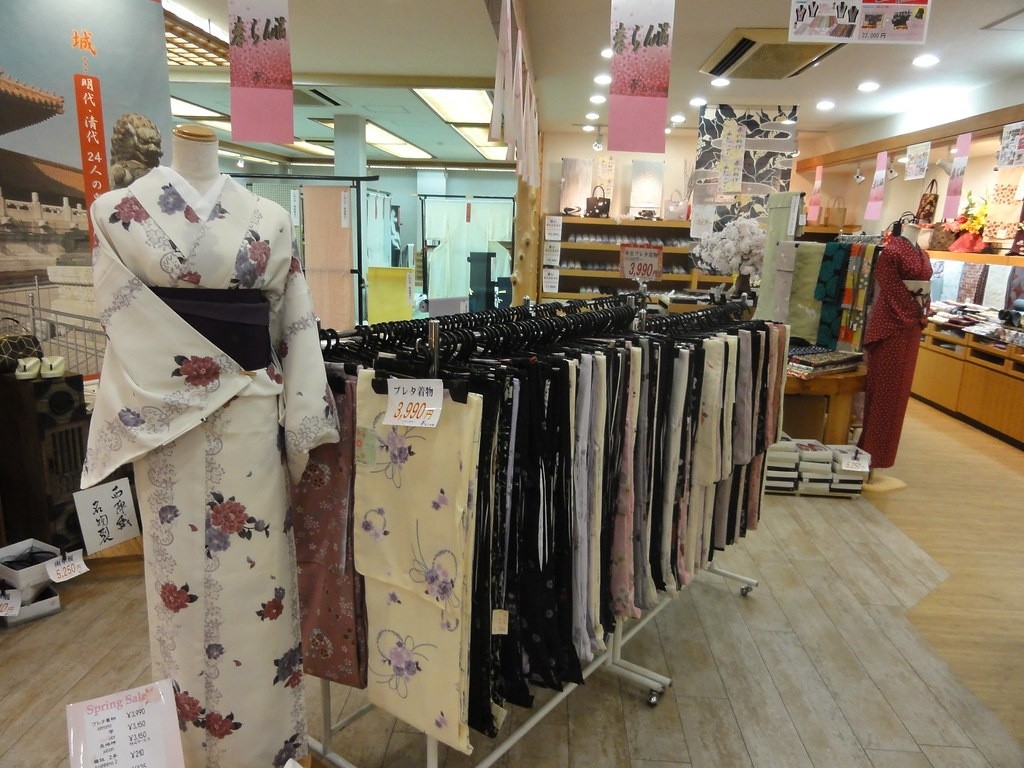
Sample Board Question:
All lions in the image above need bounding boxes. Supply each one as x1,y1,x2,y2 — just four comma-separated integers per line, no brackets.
108,112,165,191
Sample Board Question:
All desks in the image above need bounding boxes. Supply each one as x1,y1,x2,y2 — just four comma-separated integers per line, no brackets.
784,363,866,444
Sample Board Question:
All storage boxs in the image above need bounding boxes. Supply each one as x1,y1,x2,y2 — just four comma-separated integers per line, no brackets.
0,537,60,630
760,436,872,501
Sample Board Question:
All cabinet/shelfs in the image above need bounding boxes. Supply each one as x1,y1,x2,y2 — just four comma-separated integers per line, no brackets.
957,332,1024,443
907,320,970,412
539,212,755,321
0,370,89,553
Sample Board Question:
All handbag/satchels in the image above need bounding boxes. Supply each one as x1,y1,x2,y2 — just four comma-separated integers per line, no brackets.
584,186,610,218
915,179,939,224
663,190,689,222
882,212,956,251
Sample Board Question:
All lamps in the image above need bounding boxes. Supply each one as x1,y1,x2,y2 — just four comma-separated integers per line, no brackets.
591,126,603,153
853,131,1003,184
236,154,244,167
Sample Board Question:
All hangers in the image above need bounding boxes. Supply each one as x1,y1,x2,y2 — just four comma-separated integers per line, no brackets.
316,288,756,406
833,235,876,245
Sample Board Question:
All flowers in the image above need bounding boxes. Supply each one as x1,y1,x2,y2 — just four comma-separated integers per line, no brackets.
688,216,764,278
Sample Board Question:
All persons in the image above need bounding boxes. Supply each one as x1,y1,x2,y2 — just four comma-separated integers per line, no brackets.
863,223,933,475
80,126,341,768
